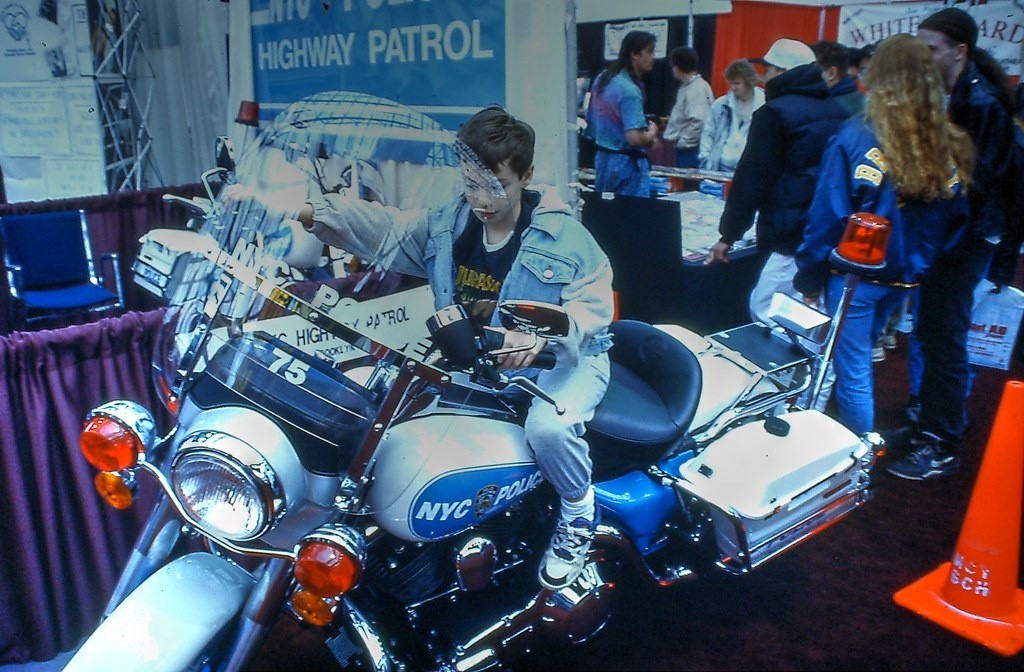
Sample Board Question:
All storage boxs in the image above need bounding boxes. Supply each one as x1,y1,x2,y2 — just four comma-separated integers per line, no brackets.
678,410,866,564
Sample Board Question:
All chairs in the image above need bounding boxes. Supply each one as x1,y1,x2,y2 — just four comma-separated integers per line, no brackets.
0,207,127,331
581,190,682,328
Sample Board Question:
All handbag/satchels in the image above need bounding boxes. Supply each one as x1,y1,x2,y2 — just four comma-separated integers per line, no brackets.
966,279,1024,371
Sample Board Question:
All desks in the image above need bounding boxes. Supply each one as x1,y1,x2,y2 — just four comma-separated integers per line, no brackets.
652,187,758,333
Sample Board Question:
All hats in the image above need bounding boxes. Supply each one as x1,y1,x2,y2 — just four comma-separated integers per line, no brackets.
748,37,818,70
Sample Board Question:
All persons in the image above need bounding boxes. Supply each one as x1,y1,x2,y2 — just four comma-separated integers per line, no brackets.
807,38,888,119
218,105,614,593
791,31,976,439
887,7,1012,482
696,59,766,202
661,46,715,170
704,38,852,413
973,45,1024,291
583,29,658,199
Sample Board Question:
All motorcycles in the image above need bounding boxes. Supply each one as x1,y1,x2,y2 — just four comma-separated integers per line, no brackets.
44,87,888,672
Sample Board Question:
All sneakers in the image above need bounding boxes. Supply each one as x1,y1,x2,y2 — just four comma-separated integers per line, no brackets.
880,422,917,454
885,444,962,481
870,340,886,362
875,336,897,349
537,495,602,592
888,314,916,333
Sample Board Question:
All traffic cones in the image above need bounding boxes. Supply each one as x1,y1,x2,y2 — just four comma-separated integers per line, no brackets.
891,378,1024,658
